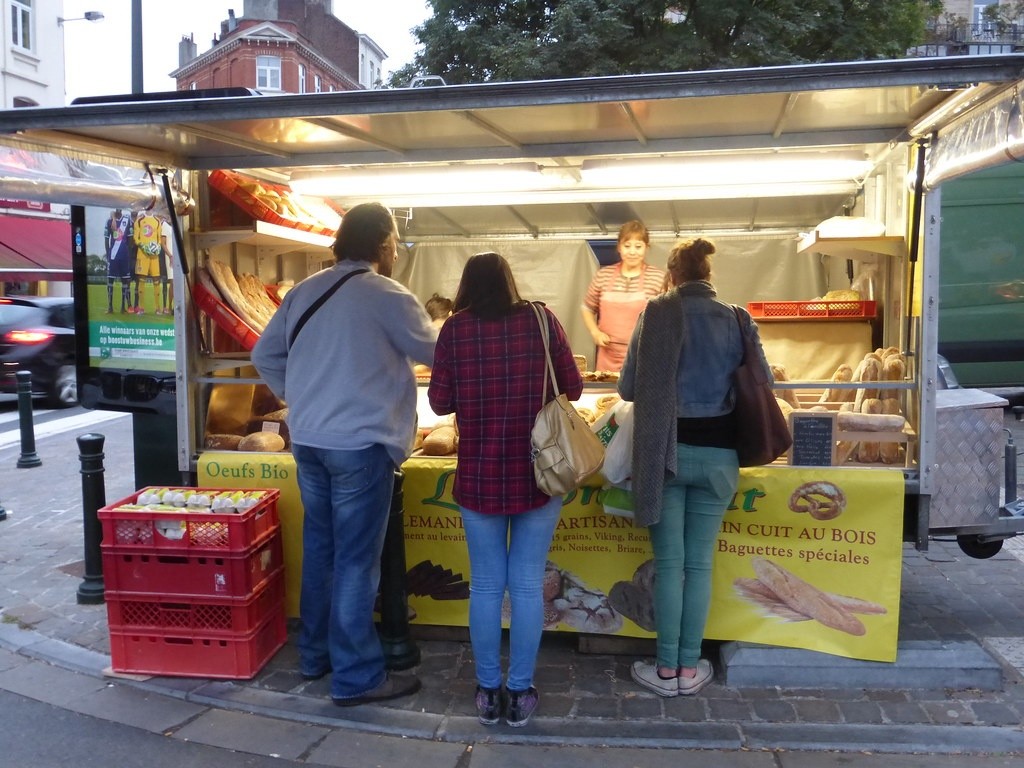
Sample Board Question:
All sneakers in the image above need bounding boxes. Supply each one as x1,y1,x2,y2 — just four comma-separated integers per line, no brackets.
331,673,421,706
678,659,713,695
506,684,539,727
630,659,679,697
475,685,502,724
299,672,324,681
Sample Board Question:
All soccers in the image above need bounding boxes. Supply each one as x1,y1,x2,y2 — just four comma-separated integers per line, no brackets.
146,241,161,256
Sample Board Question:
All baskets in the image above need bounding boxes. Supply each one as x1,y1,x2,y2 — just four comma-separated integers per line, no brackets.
748,300,879,321
194,280,279,352
276,183,346,238
209,167,325,235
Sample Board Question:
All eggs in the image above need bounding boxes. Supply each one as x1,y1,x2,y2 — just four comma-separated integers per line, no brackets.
136,491,259,538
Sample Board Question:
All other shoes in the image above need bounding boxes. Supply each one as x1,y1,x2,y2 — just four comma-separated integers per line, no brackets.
164,309,170,313
121,310,128,314
105,308,113,314
138,309,144,315
155,310,162,314
127,306,134,313
134,306,140,314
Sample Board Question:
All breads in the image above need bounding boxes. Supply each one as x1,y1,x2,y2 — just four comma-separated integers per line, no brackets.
818,289,862,311
205,407,292,453
409,364,459,456
570,355,620,427
406,560,471,600
495,557,658,635
733,556,887,636
769,346,908,464
206,177,340,337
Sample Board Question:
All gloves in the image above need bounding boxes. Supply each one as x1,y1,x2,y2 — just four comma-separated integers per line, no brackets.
140,243,148,253
170,256,173,264
156,243,162,255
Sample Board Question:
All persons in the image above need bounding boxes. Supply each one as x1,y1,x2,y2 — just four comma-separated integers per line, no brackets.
581,220,676,372
250,200,440,708
427,253,585,727
614,238,775,698
103,209,174,315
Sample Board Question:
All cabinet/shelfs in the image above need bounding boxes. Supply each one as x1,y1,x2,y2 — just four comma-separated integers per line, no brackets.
190,220,338,375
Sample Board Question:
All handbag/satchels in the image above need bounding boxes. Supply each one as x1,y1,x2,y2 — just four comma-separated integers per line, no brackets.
589,400,633,484
529,300,607,497
733,305,793,468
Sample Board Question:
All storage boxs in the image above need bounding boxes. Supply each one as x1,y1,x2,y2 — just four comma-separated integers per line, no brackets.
193,282,282,351
106,579,290,679
98,485,283,602
929,388,1009,529
748,300,878,323
207,170,344,238
